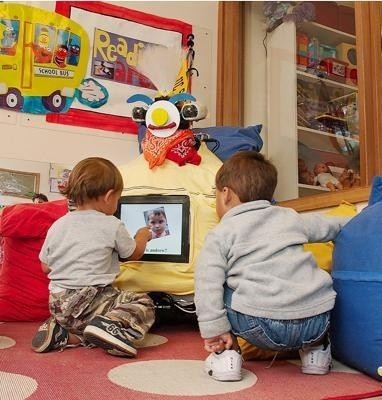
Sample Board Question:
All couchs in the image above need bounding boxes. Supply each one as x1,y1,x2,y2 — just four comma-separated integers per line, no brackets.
1,195,69,323
328,176,381,381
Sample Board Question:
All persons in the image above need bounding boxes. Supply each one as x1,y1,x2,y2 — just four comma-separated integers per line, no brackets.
192,151,356,383
32,156,158,357
142,210,168,238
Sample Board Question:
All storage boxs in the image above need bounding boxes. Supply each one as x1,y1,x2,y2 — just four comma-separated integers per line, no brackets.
310,2,340,36
336,5,356,36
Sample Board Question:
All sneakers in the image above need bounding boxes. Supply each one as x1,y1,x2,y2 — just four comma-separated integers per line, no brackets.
298,329,333,376
83,315,138,358
202,348,244,382
31,314,70,353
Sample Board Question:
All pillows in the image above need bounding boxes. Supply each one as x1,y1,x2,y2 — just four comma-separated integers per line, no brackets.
136,122,265,170
301,199,360,272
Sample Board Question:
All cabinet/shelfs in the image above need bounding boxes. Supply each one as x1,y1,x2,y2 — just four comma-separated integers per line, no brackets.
264,17,366,202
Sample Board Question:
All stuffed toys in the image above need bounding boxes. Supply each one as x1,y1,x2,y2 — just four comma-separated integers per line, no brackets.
126,93,222,169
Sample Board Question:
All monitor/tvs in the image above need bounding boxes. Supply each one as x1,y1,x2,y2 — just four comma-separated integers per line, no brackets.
112,195,189,262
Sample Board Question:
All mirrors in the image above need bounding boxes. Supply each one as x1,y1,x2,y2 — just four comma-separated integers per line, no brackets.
0,166,41,200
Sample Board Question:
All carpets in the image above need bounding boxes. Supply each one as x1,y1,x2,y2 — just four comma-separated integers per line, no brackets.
0,318,382,400
